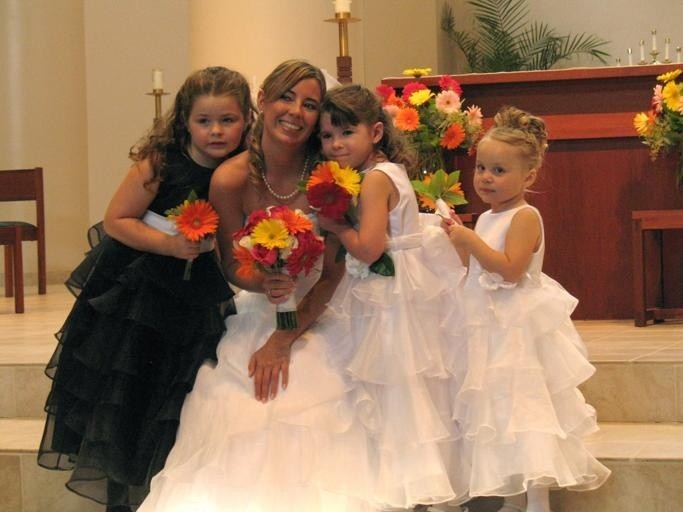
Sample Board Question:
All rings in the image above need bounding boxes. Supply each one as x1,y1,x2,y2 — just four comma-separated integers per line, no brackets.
266,288,272,300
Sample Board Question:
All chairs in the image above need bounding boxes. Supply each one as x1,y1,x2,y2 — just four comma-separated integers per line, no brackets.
1,166,48,316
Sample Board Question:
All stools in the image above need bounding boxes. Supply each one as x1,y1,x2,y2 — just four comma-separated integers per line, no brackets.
632,206,682,326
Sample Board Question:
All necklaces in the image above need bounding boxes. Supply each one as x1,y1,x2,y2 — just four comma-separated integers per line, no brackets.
255,155,310,200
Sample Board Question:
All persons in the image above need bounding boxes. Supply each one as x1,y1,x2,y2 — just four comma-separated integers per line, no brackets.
34,65,261,512
317,80,480,512
441,107,613,512
135,57,457,512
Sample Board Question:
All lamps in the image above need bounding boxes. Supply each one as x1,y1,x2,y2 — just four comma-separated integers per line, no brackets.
614,25,682,68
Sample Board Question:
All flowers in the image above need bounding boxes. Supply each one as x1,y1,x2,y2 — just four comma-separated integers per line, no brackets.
375,64,484,230
298,159,396,278
233,205,326,331
631,68,683,176
162,190,219,286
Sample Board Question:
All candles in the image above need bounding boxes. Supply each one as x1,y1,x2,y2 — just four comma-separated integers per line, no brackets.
151,69,163,92
331,0,353,14
